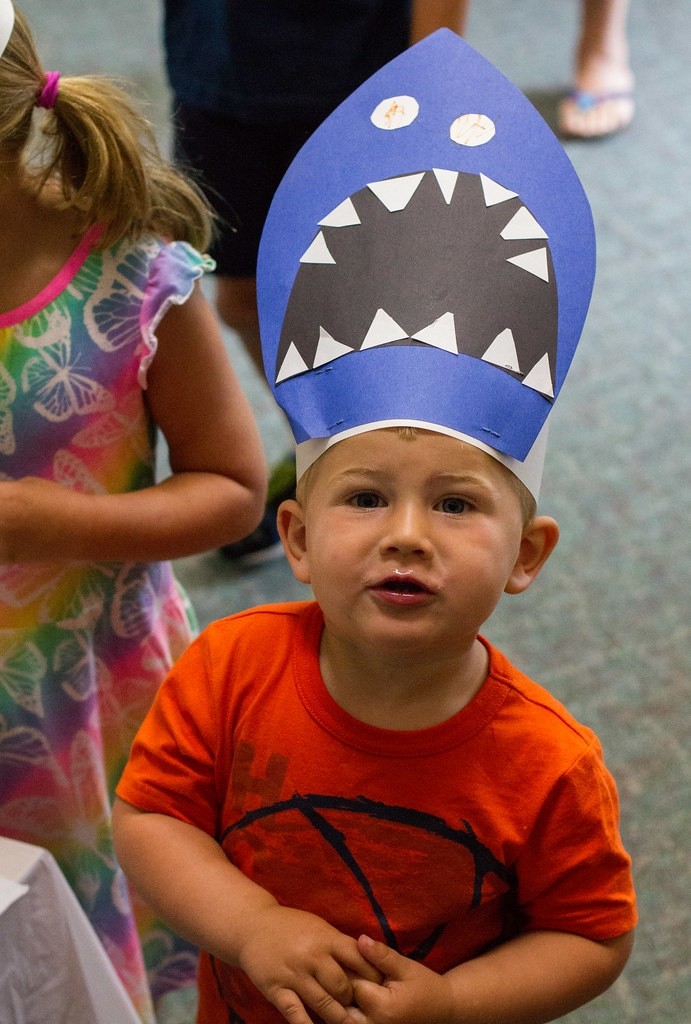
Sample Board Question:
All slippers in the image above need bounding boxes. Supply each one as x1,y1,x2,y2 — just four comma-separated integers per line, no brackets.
557,86,639,140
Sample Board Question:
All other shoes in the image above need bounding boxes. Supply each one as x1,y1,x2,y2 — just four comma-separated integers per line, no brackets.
222,446,297,566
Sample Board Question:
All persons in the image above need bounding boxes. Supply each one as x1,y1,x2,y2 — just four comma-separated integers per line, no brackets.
163,2,635,566
113,364,635,1024
0,0,267,1023
